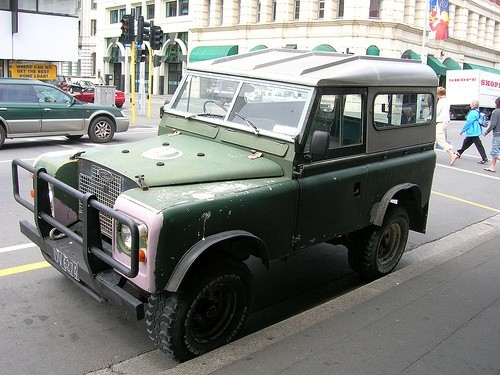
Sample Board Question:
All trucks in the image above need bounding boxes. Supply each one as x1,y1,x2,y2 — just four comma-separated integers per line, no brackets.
445,68,500,121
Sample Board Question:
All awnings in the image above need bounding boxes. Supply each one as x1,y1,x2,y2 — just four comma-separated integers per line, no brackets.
401,50,421,60
427,54,448,77
249,45,268,52
366,45,379,57
312,44,337,52
463,62,500,75
443,57,461,69
282,44,297,49
189,45,238,63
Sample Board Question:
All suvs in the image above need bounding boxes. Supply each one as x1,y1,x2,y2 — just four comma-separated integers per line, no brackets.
11,49,441,361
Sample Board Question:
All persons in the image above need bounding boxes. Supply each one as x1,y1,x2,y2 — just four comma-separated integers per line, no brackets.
483,96,500,173
434,86,459,166
451,99,488,164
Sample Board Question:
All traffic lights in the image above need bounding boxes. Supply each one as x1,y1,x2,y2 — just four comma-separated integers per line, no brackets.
138,15,152,46
149,25,166,50
119,13,135,44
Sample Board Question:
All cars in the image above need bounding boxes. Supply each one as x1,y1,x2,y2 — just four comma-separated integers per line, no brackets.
74,85,125,107
0,78,130,149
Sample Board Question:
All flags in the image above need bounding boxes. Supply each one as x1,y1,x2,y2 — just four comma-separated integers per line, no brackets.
430,0,449,40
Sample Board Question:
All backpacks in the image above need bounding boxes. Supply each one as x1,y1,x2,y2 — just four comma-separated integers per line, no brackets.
473,109,486,127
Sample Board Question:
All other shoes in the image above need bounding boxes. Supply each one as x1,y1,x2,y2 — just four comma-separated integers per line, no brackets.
450,155,459,165
478,158,489,164
484,168,496,172
454,152,461,158
